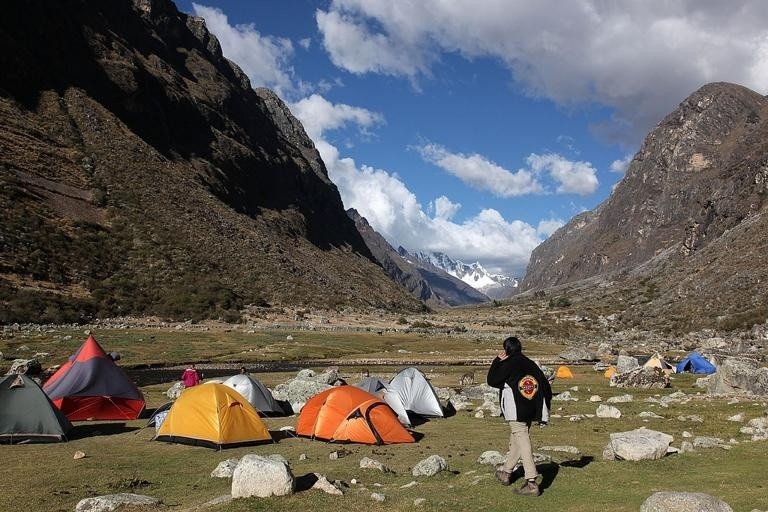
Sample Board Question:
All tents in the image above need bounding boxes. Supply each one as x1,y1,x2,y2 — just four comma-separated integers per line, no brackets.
222,373,286,417
0,372,74,443
676,351,716,374
643,351,677,374
387,367,445,418
148,382,277,451
296,384,417,444
354,376,415,428
41,334,147,421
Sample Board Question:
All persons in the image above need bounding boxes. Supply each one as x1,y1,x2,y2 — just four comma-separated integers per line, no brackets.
240,366,250,376
180,363,200,388
486,336,553,497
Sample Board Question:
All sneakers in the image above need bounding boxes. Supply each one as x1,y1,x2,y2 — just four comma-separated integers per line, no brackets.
512,481,540,496
496,469,511,485
